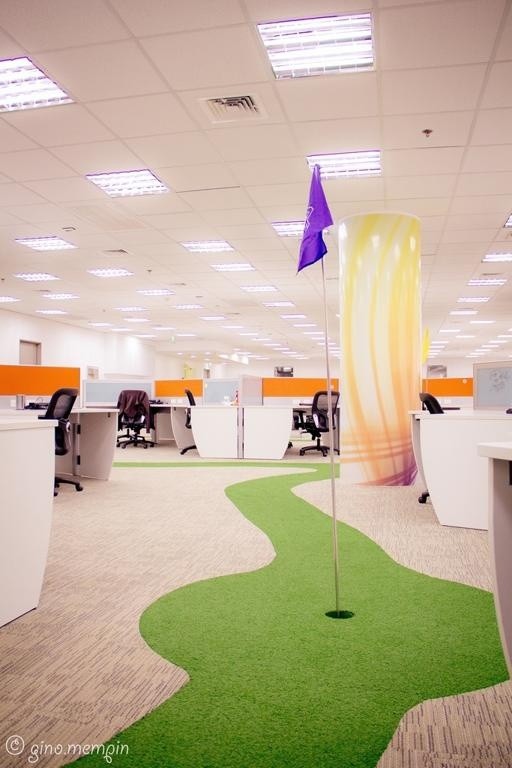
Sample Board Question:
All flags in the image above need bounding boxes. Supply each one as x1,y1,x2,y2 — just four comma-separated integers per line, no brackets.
296,164,334,272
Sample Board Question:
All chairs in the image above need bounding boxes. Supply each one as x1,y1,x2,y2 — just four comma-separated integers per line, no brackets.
295,391,341,456
418,394,460,503
180,389,197,454
117,390,155,449
38,388,83,495
